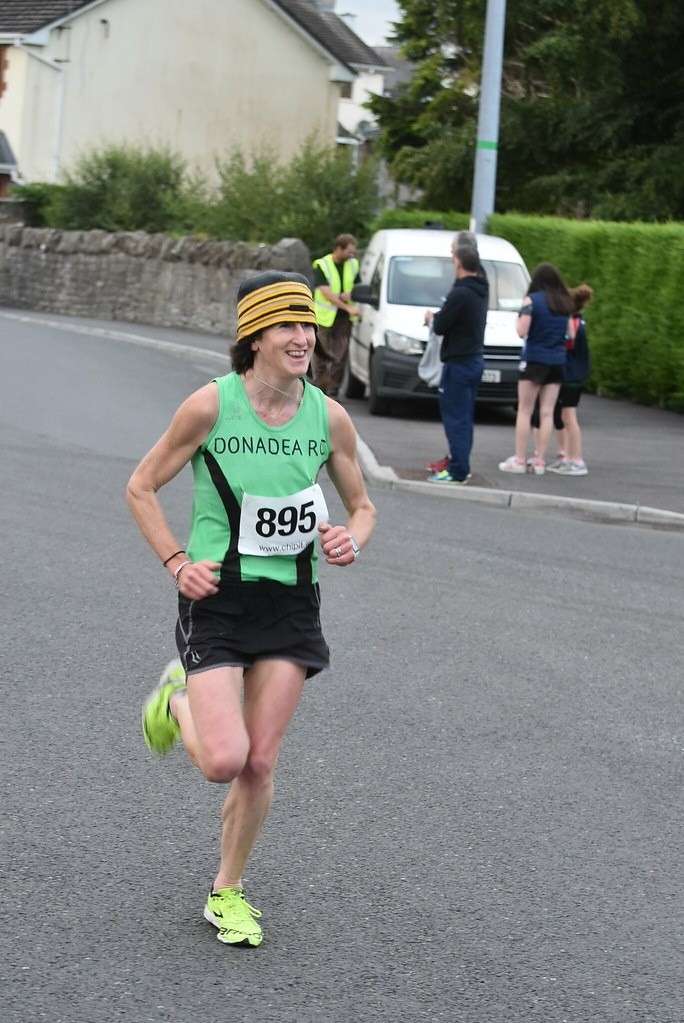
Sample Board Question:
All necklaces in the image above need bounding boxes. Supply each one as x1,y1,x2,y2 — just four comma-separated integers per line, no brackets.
255,377,302,405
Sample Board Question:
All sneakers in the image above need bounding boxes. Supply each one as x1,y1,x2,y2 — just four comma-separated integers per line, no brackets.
425,455,450,473
498,455,526,473
546,452,588,476
203,882,263,948
526,458,546,474
142,657,186,761
427,470,472,485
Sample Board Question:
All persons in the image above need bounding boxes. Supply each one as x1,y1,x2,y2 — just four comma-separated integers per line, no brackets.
498,262,575,477
425,231,489,486
311,233,361,405
126,269,377,950
549,285,593,476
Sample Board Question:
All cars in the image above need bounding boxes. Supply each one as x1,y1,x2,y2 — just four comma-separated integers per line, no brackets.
340,228,559,410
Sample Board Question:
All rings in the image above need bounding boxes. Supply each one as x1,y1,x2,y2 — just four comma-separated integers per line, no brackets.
335,548,342,557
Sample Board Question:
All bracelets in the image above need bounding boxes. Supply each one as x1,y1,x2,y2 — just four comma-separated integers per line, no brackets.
164,550,186,567
348,535,361,565
173,560,193,591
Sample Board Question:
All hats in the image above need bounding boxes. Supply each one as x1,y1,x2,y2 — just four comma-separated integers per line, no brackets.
235,271,318,344
